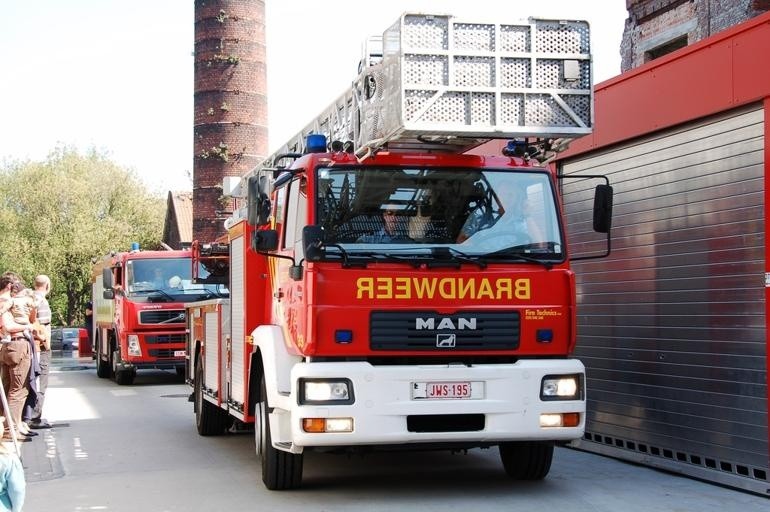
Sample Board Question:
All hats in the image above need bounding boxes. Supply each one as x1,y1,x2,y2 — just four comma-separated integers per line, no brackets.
11,283,25,293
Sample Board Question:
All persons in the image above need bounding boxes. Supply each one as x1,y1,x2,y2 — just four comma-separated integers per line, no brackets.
356,200,421,258
0,270,54,443
457,181,544,252
84,292,94,350
148,267,170,289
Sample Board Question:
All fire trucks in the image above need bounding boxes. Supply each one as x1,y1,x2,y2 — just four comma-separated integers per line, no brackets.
91,244,231,386
186,15,613,491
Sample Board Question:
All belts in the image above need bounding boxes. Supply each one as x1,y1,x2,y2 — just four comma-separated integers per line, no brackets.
11,336,28,340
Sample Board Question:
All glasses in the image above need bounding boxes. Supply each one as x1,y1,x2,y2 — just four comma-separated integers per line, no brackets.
385,210,402,216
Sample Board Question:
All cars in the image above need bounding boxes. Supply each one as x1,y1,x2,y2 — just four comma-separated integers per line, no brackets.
51,327,79,351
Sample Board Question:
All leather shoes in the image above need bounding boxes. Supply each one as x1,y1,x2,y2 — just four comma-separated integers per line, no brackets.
2,423,51,442
